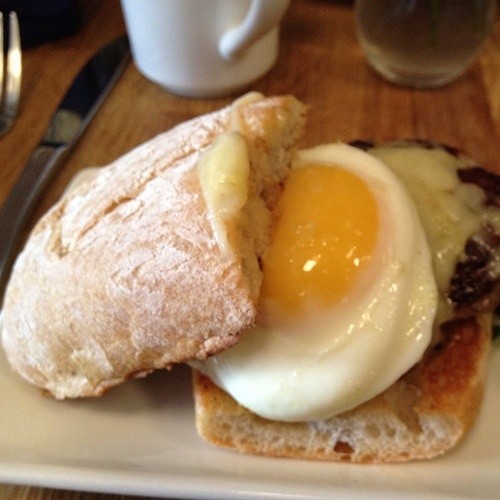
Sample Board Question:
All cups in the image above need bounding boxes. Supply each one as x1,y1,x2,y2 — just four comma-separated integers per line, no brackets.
119,0,291,98
0,12,23,137
354,0,490,87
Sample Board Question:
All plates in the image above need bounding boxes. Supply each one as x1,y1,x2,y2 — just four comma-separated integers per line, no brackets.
0,166,499,500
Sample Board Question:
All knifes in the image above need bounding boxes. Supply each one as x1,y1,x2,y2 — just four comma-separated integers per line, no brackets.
0,33,132,273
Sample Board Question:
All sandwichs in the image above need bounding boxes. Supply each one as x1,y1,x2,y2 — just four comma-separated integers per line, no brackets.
0,89,500,464
